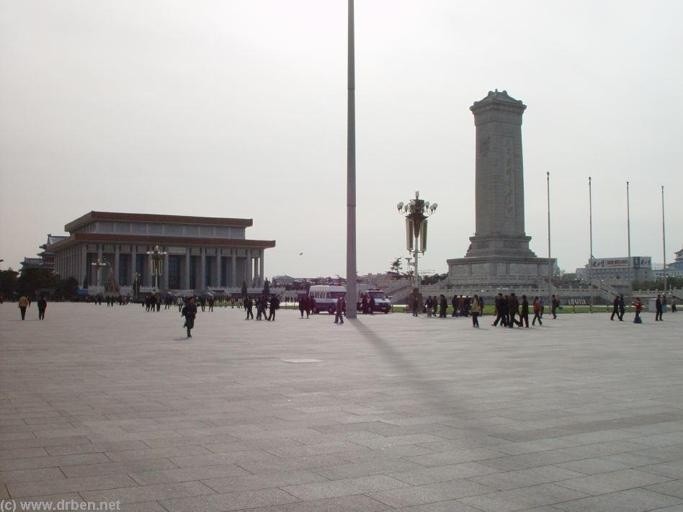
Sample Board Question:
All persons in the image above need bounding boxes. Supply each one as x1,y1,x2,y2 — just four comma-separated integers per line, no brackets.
470,294,480,327
81,291,243,312
17,293,29,320
619,293,624,320
489,290,546,328
180,295,197,337
631,296,641,323
367,295,374,314
423,294,483,318
37,294,46,320
551,294,558,320
333,293,343,324
610,295,621,321
655,294,663,321
341,295,346,315
243,292,315,322
411,294,418,317
361,295,367,314
671,295,679,312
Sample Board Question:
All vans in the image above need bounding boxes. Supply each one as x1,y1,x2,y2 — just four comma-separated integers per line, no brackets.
362,288,391,313
308,285,346,315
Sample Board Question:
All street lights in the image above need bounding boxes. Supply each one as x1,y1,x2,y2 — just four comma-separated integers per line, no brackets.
131,272,141,299
91,258,105,287
147,246,167,291
395,190,437,290
